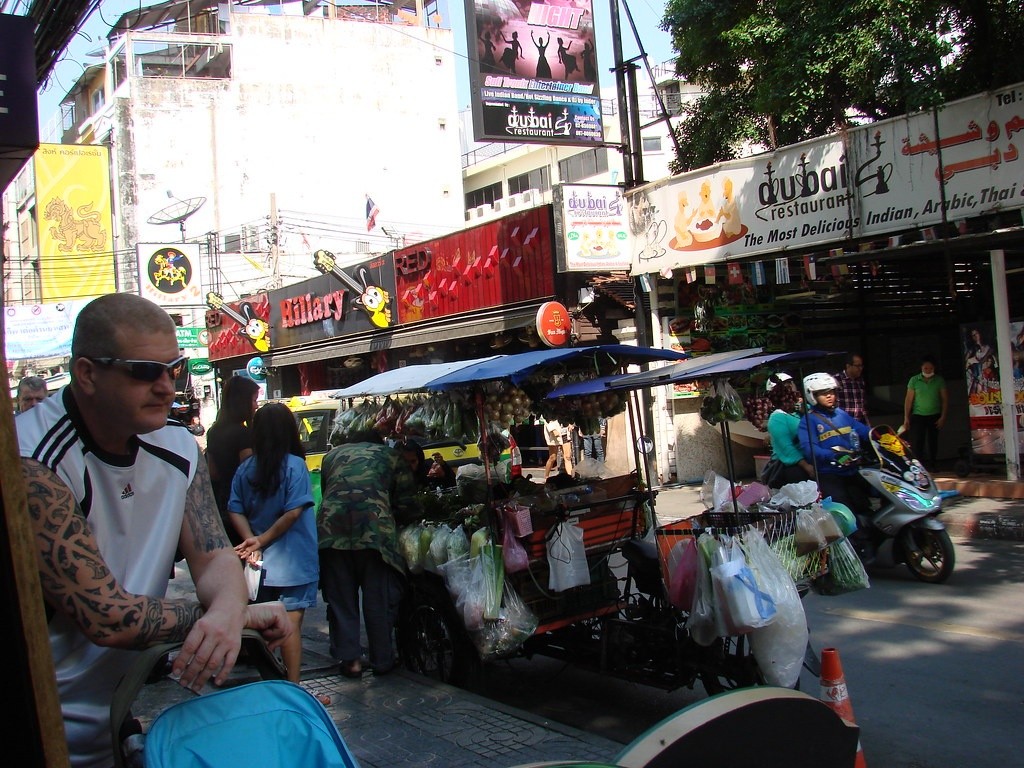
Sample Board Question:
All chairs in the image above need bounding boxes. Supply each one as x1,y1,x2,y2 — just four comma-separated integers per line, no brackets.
309,430,320,452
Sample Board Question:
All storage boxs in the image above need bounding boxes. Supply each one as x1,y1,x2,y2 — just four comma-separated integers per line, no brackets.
654,509,798,591
753,454,772,481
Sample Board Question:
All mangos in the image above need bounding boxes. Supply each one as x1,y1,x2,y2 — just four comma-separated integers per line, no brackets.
700,395,745,424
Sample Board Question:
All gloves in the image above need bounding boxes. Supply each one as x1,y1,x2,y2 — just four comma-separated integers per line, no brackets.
836,454,853,466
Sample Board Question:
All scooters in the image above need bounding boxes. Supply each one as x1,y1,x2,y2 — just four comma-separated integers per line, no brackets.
830,423,956,583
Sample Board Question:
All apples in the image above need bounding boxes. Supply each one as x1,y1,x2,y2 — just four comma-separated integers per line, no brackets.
554,393,619,418
483,384,533,429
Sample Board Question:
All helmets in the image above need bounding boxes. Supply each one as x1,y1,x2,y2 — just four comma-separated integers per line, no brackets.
803,373,838,406
766,373,793,389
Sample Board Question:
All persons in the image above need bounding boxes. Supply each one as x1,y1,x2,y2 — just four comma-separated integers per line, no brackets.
16,293,293,768
227,403,330,706
206,376,261,547
903,355,948,464
316,431,417,677
543,419,573,483
761,373,815,489
394,438,456,488
15,377,47,417
831,352,865,420
796,373,910,498
579,418,606,462
192,415,200,425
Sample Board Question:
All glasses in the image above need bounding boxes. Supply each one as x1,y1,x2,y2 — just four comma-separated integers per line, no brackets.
78,354,187,382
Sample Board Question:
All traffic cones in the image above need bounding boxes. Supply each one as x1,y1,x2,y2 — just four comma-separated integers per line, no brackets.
815,645,870,768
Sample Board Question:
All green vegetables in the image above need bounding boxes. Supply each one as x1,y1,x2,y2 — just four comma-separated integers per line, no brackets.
415,490,458,525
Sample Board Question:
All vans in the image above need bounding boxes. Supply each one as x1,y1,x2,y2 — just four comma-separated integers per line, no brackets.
279,396,523,527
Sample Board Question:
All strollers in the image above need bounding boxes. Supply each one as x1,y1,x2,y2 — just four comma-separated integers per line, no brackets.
115,630,360,768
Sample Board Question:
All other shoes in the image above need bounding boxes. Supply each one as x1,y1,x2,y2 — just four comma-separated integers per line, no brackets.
928,467,939,473
308,691,332,705
338,661,363,678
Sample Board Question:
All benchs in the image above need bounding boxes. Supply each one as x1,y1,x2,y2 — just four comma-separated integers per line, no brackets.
522,492,640,562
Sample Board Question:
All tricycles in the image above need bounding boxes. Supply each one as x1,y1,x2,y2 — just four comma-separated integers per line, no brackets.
327,346,802,701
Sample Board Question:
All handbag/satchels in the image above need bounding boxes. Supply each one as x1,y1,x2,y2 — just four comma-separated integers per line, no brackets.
330,386,529,447
397,519,541,660
668,527,781,646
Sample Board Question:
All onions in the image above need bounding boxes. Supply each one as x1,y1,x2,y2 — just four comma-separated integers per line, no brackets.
725,388,795,413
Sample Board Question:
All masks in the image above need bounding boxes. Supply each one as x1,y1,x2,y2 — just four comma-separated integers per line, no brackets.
922,370,935,378
